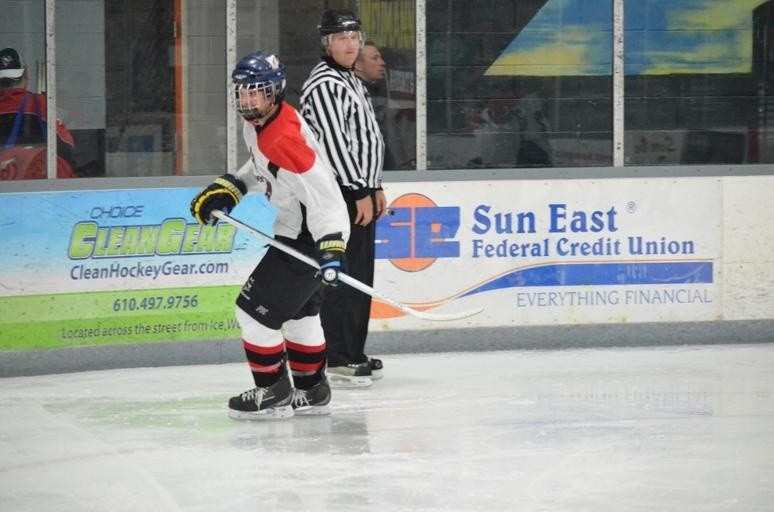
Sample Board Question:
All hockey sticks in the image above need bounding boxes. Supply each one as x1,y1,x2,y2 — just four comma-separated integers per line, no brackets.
212,209,484,321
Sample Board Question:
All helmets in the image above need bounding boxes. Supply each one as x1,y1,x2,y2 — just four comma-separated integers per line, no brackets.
229,48,288,101
316,7,365,36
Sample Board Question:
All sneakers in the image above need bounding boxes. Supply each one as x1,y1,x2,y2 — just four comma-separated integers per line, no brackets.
322,354,387,390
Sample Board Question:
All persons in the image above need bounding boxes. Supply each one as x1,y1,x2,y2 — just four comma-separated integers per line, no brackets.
297,6,386,385
455,27,553,167
0,46,75,179
189,49,353,417
350,42,389,126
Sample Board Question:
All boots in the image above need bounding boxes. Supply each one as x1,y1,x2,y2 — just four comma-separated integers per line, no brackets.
225,354,295,423
290,355,335,418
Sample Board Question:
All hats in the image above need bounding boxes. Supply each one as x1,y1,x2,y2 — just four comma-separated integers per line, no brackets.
0,47,26,81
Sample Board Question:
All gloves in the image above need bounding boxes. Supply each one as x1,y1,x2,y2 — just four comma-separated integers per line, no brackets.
312,231,350,291
188,171,249,229
